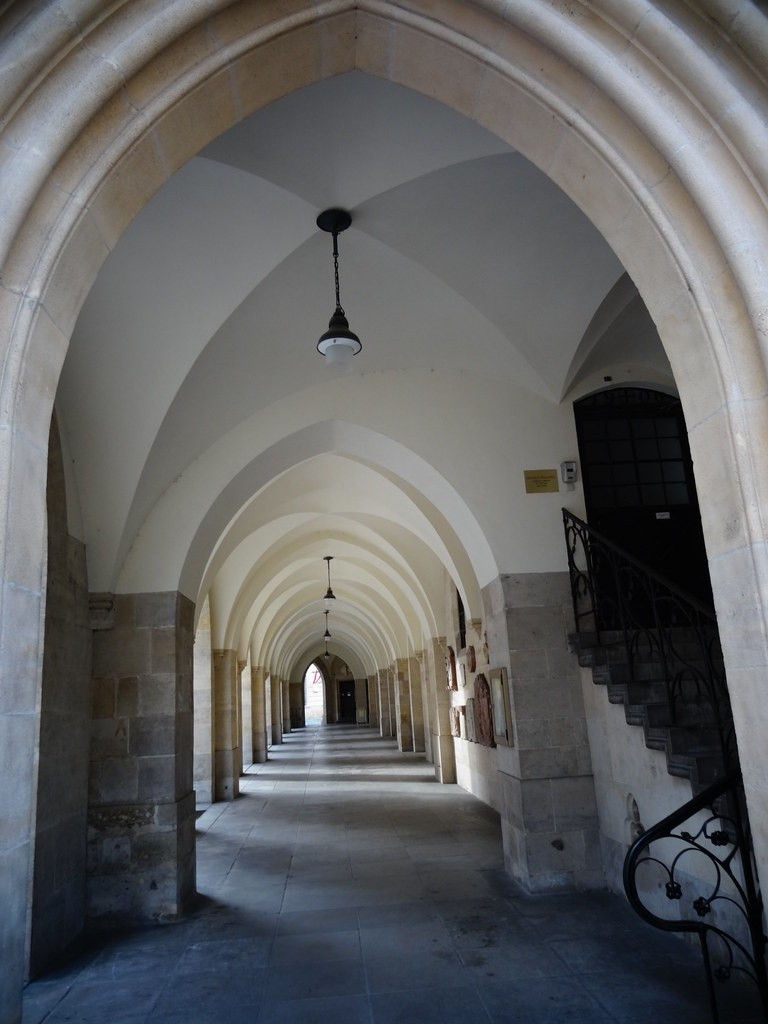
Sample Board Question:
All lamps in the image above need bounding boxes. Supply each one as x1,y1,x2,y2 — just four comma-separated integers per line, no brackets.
323,611,331,642
325,642,330,659
316,207,363,369
322,556,336,609
